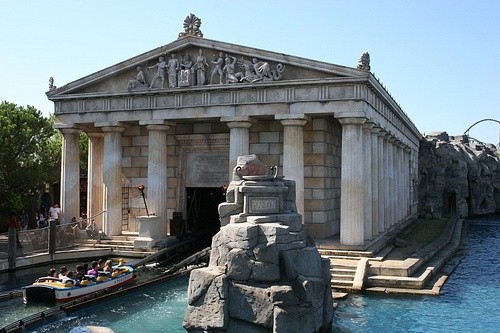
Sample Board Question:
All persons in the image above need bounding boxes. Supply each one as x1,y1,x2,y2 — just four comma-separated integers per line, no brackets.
75,265,86,282
147,56,165,89
55,225,66,247
82,263,89,275
58,266,76,285
193,49,209,85
36,210,48,228
47,267,57,279
127,65,146,90
67,271,80,285
29,209,37,229
9,209,19,229
181,53,192,83
87,261,100,278
85,219,99,239
49,202,60,225
103,260,117,274
253,58,267,77
223,56,243,84
20,209,28,231
209,52,223,84
70,216,79,237
166,53,179,88
97,259,105,271
80,207,87,223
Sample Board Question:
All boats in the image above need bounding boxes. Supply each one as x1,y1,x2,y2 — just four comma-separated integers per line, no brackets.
21,264,135,302
